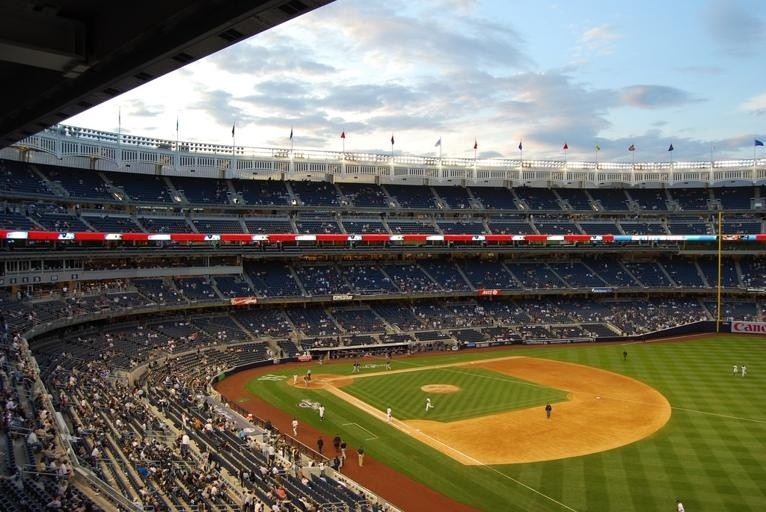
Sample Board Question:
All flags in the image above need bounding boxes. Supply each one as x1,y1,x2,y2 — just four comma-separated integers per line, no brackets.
232,125,235,138
290,129,293,140
629,145,635,151
391,136,395,144
341,132,345,138
434,138,440,147
474,137,478,149
176,121,178,132
595,144,600,152
119,111,120,123
755,140,763,146
668,144,673,151
563,144,568,149
519,142,522,150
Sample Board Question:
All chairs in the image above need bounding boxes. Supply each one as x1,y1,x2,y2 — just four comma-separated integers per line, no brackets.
381,185,766,344
0,159,382,511
